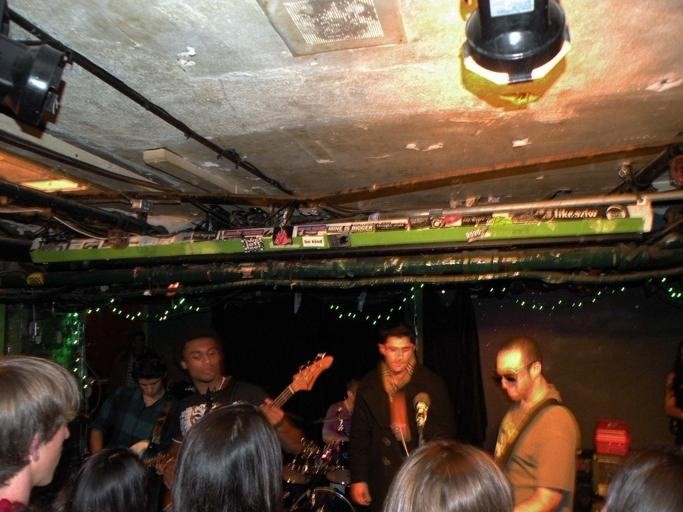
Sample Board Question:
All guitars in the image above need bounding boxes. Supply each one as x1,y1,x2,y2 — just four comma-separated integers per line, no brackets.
83,438,150,457
159,352,334,512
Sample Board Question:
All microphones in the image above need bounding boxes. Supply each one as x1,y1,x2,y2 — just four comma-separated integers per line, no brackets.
413,390,432,431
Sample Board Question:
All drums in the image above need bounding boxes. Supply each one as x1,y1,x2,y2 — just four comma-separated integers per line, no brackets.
281,437,323,484
321,439,352,486
282,487,355,511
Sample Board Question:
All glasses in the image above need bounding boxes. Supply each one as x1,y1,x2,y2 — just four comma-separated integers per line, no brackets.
493,360,538,388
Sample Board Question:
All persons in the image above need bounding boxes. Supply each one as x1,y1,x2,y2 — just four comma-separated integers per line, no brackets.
86,354,177,454
345,322,452,512
321,377,357,446
55,448,160,511
0,356,81,511
382,437,514,512
664,363,682,443
491,335,581,511
171,401,283,512
156,327,305,488
599,447,683,510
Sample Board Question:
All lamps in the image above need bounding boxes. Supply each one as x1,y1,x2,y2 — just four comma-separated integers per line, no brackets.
0,33,65,132
461,1,575,87
143,148,249,194
